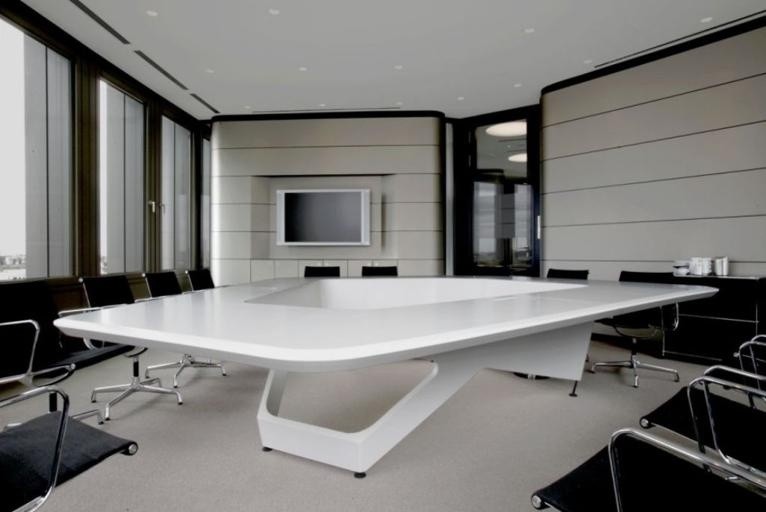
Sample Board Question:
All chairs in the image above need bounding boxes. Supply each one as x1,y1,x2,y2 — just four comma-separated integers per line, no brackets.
1,258,766,512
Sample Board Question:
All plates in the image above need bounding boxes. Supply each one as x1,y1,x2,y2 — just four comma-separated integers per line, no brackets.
692,256,713,276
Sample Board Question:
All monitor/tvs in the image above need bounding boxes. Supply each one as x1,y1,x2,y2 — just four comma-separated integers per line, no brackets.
275,188,371,248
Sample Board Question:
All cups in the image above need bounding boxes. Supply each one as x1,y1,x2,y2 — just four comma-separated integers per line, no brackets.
714,257,729,275
672,265,690,276
